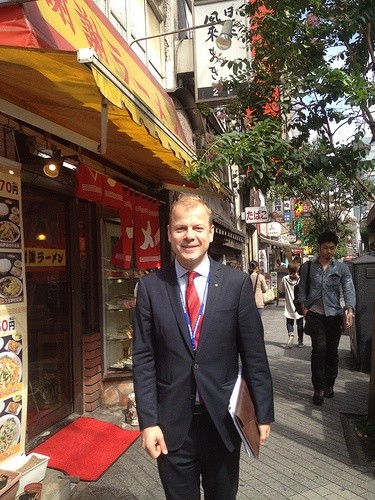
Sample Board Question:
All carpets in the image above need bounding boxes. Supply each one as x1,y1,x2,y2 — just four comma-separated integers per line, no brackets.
25,416,143,483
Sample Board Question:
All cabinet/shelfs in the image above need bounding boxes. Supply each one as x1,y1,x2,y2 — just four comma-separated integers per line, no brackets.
102,274,137,379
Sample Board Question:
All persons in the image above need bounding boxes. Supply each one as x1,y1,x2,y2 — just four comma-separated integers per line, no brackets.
246,261,268,317
276,258,286,268
296,232,357,404
278,262,305,349
131,194,275,500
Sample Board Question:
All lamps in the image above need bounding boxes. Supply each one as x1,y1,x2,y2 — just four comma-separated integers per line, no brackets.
130,19,234,50
42,149,65,178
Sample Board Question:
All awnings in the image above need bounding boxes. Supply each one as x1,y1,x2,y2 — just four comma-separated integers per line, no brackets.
0,35,201,199
259,234,292,249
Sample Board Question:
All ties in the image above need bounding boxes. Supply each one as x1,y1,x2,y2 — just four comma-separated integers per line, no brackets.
184,270,201,332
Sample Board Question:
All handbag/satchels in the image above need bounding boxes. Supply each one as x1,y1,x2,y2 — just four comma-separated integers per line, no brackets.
292,261,311,315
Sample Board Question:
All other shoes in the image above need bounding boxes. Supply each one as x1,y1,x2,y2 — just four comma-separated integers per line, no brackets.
286,334,294,348
312,388,324,404
298,343,304,347
326,385,334,397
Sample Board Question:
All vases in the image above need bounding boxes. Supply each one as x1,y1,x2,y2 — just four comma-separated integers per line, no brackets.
0,452,51,500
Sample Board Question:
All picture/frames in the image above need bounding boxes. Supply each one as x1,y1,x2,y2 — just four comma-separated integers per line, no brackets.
102,219,121,268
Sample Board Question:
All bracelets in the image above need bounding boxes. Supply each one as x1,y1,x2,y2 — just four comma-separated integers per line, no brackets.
347,312,355,319
